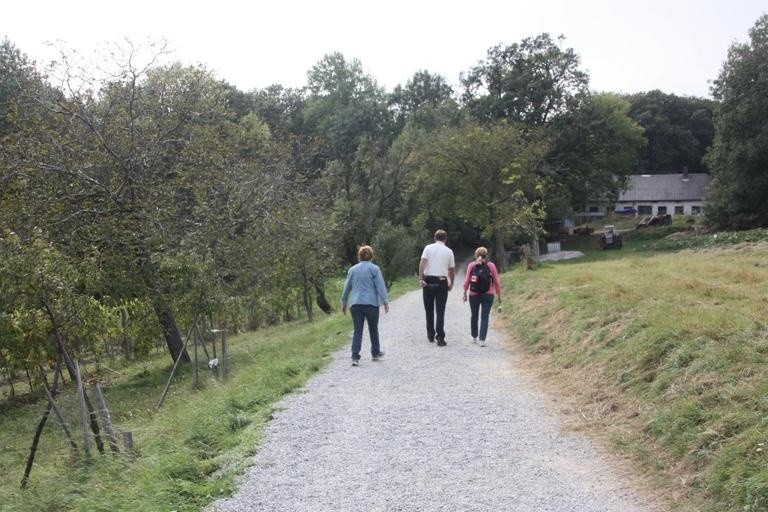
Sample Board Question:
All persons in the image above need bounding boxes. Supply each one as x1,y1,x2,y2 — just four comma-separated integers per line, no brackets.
341,244,390,367
419,228,456,346
463,246,501,348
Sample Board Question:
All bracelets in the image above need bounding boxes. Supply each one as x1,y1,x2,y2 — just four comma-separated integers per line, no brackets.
419,278,424,280
463,291,467,296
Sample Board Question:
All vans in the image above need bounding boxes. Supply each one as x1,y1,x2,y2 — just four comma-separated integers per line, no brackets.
636,214,671,229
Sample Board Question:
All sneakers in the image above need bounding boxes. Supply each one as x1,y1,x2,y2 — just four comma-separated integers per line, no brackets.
351,359,358,365
473,337,485,347
372,352,383,361
438,339,447,346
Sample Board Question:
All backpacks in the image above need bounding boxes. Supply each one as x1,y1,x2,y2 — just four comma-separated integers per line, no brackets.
471,262,491,293
